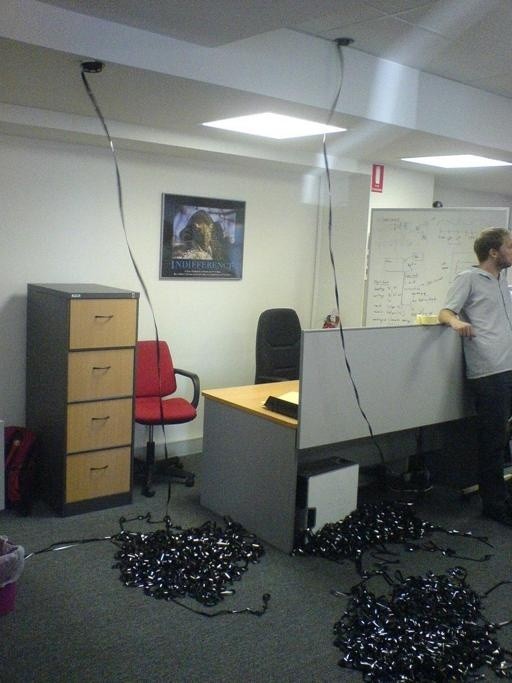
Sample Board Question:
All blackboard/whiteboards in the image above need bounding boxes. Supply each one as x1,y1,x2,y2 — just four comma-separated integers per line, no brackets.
363,207,510,328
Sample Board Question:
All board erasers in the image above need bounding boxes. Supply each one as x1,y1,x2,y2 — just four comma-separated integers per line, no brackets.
416,314,438,325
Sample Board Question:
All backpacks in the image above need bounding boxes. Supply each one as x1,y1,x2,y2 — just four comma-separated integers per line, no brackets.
5,426,49,516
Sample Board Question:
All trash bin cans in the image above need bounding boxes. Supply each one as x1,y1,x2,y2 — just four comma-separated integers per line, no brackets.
0,535,24,617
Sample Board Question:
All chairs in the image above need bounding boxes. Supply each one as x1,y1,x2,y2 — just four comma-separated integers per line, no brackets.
254,307,303,384
134,340,200,497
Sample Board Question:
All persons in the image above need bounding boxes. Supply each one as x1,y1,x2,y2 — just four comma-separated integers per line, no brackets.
439,227,512,526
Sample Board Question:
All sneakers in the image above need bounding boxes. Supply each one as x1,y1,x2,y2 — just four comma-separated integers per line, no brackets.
482,497,511,527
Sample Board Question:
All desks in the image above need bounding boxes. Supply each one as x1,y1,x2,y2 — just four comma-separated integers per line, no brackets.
200,379,304,557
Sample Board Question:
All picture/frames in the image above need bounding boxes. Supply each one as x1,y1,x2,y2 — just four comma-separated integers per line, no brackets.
158,192,246,281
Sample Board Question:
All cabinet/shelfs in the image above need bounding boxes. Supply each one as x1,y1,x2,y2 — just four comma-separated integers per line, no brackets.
25,283,141,518
